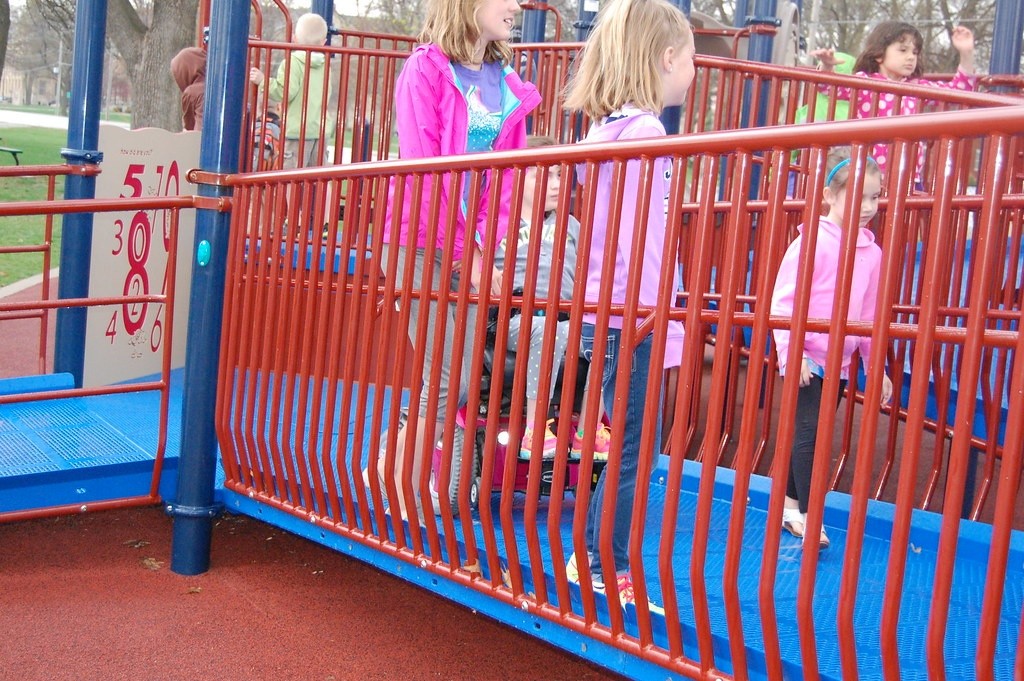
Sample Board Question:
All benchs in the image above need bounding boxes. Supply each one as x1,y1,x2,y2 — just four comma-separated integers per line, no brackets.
0,147,23,165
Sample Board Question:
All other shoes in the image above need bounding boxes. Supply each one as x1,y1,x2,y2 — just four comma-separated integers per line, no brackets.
602,570,666,616
385,506,426,529
363,468,388,499
782,508,805,537
802,525,829,548
270,219,301,238
322,223,328,238
566,552,606,594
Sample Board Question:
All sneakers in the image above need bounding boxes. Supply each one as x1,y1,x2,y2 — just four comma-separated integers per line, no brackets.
520,419,570,460
571,423,611,461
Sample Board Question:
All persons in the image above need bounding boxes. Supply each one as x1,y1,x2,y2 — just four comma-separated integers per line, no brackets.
809,20,977,247
761,141,892,551
246,87,282,191
470,134,619,462
249,12,334,238
360,1,543,523
561,1,697,618
170,46,208,133
